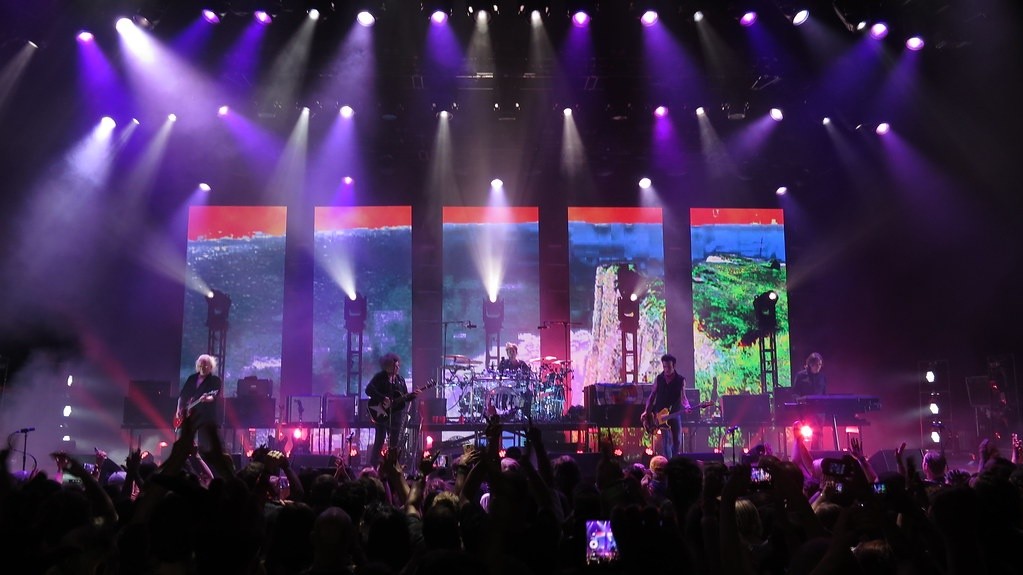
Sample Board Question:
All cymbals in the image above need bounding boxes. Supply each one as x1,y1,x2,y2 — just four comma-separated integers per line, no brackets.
529,356,557,363
552,360,573,365
439,354,483,370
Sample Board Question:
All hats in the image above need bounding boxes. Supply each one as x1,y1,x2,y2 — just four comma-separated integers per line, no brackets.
649,455,668,473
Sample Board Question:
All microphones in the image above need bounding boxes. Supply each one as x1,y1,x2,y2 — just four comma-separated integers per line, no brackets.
16,427,36,433
345,432,354,443
538,326,550,329
727,426,738,433
466,325,477,328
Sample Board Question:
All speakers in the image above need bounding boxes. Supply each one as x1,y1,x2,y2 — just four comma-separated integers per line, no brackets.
419,398,446,423
549,452,601,480
286,396,322,422
678,451,725,467
224,397,275,427
323,395,356,425
358,400,376,424
126,382,171,424
717,394,770,425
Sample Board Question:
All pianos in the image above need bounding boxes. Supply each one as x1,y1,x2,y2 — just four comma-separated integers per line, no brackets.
776,394,884,461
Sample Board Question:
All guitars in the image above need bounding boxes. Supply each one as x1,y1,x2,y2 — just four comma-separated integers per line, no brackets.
173,389,219,429
642,401,715,435
367,378,436,424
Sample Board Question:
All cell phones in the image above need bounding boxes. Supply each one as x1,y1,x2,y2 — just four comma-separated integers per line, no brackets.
84,464,96,474
276,477,290,488
874,482,886,494
750,467,773,485
463,443,474,454
585,520,619,564
821,458,850,477
833,483,844,495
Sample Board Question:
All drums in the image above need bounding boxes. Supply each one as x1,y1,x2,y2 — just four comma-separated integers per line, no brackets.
485,386,520,420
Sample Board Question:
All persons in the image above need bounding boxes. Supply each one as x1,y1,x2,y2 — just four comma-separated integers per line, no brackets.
641,355,691,459
176,354,221,459
498,344,532,419
365,353,422,470
0,421,1023,575
791,352,827,465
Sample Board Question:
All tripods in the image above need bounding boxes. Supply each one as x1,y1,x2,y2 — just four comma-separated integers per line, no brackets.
532,361,571,424
